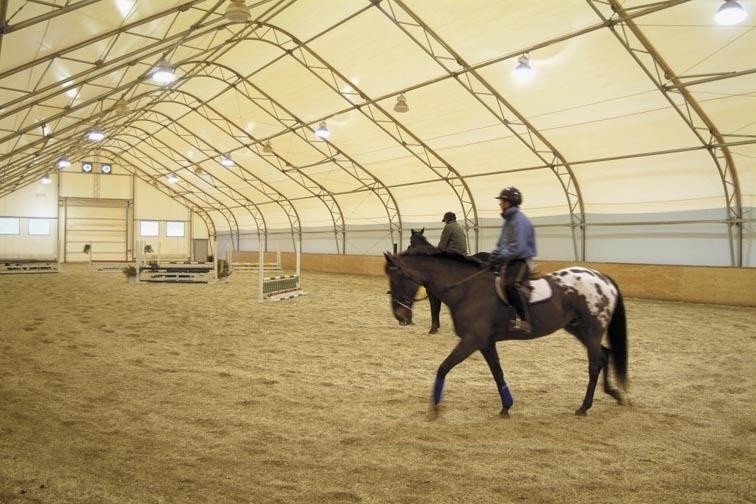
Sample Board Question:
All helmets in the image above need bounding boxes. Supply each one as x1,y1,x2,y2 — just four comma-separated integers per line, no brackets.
495,188,521,206
442,212,456,222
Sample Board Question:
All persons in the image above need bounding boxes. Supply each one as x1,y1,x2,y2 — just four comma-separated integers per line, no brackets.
436,212,466,256
486,187,536,334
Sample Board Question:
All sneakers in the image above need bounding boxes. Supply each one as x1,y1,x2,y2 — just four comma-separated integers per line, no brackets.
507,319,531,335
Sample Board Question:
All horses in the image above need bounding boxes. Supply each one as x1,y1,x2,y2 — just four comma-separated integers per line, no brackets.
407,226,499,335
381,246,632,424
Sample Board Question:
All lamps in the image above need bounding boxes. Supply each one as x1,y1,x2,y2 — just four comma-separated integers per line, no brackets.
223,1,252,23
714,1,747,26
151,60,175,82
392,93,410,114
263,138,272,153
